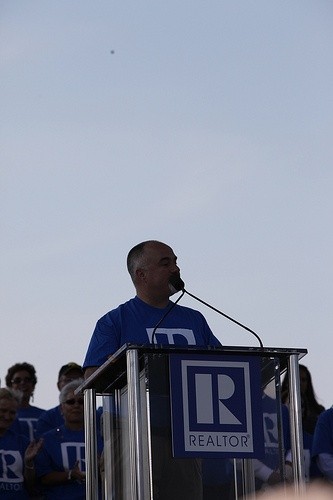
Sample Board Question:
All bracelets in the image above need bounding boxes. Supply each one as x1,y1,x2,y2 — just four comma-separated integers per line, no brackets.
68,469,72,480
23,457,37,471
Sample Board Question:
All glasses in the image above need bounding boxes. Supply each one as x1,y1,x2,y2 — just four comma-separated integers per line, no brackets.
11,376,35,383
61,398,84,404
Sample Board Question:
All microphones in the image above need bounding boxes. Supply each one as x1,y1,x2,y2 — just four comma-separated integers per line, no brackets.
152,274,263,347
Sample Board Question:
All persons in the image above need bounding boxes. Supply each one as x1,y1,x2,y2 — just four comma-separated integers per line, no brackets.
226,363,333,500
82,240,227,381
0,362,107,500
